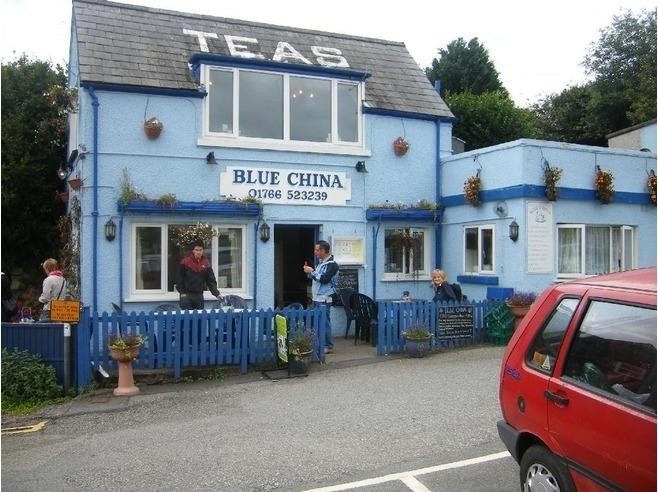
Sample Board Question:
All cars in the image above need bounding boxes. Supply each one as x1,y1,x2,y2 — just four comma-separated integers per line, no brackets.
497,267,657,492
204,247,241,276
140,254,171,272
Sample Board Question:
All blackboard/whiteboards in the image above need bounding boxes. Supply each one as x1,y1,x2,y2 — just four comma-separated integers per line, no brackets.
436,306,473,340
331,269,358,306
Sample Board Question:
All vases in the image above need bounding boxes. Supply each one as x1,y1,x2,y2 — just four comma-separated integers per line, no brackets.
510,306,531,331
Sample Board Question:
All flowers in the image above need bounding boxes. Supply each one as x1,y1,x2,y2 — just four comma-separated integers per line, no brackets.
506,288,540,306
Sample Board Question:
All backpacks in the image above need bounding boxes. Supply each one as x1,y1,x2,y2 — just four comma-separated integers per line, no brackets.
446,282,463,304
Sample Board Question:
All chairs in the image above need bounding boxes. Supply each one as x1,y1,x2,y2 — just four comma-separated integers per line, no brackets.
343,289,378,348
111,294,304,362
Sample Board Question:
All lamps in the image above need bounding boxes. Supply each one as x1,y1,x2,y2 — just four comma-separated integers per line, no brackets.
508,221,519,242
104,219,116,241
260,220,270,243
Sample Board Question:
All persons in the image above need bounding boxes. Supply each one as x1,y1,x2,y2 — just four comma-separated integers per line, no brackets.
38,258,66,322
176,241,224,310
432,269,456,300
303,240,339,354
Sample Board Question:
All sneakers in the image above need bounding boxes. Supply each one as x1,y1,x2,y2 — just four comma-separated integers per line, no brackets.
323,346,335,354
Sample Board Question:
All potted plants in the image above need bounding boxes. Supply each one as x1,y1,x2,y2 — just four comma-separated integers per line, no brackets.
288,321,318,374
399,324,433,358
108,332,148,396
